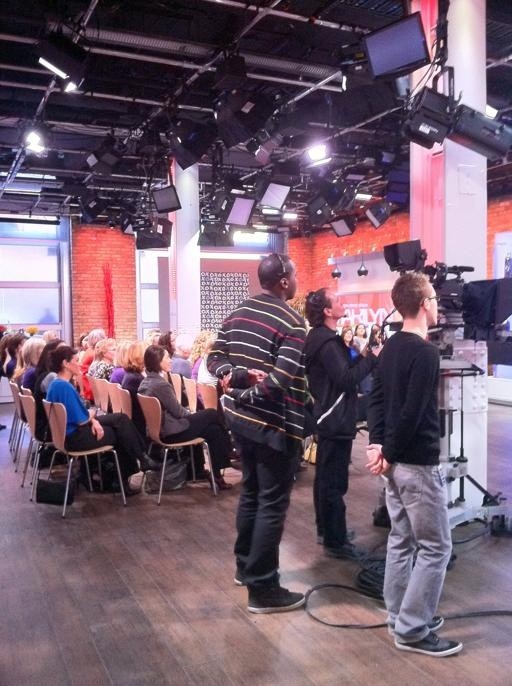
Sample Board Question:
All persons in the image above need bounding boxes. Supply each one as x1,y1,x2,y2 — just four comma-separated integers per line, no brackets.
341,323,388,396
302,288,384,561
207,253,307,613
365,273,464,657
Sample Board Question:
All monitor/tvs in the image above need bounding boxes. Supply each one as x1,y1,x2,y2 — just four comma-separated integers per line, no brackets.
361,10,431,79
150,185,181,215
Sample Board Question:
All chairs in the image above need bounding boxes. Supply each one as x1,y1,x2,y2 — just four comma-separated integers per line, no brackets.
1,366,222,522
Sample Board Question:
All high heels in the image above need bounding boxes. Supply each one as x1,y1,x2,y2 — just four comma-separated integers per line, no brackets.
140,461,163,474
208,473,232,489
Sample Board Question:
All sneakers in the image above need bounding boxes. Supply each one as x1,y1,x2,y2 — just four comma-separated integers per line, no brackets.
234,557,280,585
246,573,306,614
317,527,356,544
324,539,368,561
388,615,444,636
394,630,463,657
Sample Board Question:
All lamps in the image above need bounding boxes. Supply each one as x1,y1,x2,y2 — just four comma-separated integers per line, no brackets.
356,222,369,279
13,10,432,250
331,232,342,280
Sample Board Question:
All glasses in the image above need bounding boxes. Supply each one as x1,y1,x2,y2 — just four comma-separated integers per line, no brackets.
261,252,287,274
421,294,441,307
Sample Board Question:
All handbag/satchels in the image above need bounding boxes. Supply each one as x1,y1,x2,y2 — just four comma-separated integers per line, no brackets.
32,442,69,469
36,479,73,506
145,461,187,492
